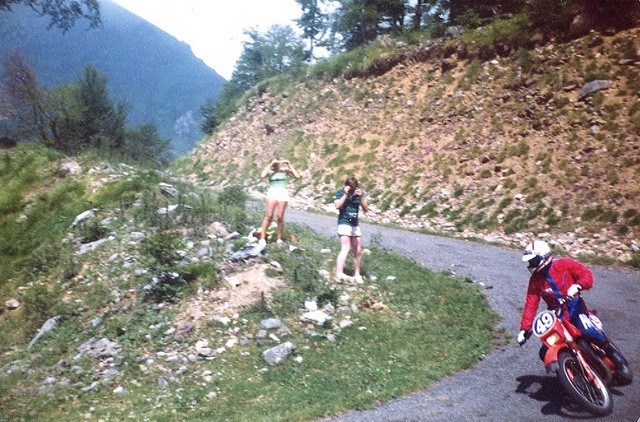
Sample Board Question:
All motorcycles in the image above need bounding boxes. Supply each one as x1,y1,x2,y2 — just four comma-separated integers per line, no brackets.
520,292,633,416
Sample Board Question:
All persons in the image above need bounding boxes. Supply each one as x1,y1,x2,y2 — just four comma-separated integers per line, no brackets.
333,176,368,284
516,241,626,386
257,158,301,252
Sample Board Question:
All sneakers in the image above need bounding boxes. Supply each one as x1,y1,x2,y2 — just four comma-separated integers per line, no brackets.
338,273,352,280
258,239,267,247
354,274,365,285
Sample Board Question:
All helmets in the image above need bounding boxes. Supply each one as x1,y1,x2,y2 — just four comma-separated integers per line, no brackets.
522,240,553,275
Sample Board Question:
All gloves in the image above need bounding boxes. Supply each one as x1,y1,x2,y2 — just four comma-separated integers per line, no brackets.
567,284,582,300
516,330,525,344
